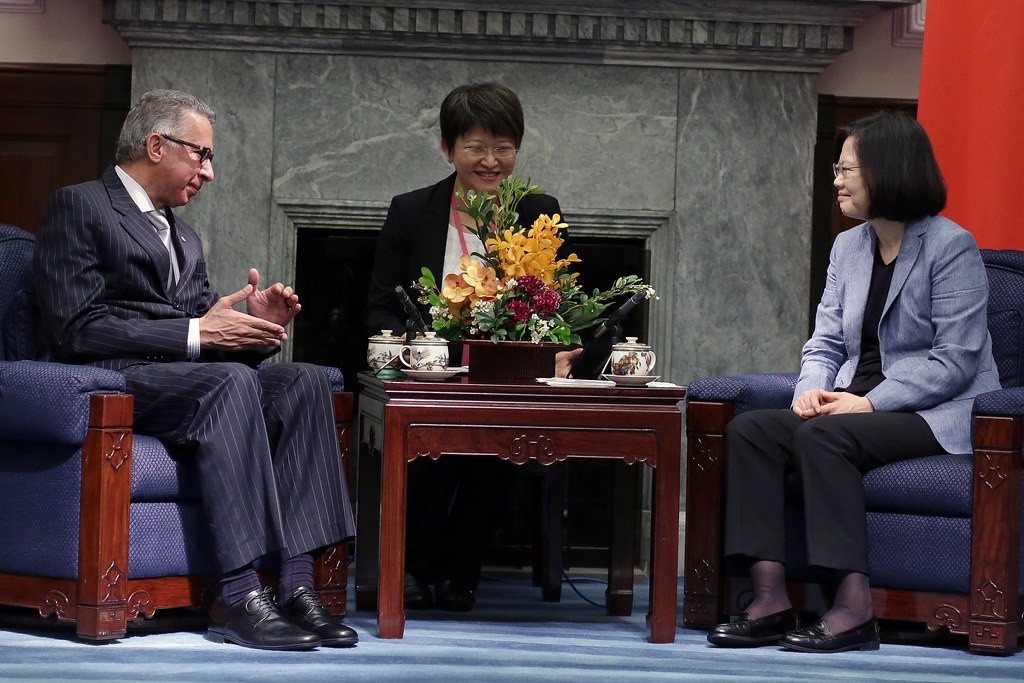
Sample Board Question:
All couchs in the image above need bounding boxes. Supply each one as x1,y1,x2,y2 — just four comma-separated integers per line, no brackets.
683,248,1024,657
0,222,352,642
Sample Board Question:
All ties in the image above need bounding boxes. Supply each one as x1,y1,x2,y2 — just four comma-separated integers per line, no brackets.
145,210,173,293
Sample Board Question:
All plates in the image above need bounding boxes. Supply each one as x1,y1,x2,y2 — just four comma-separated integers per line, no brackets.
401,370,458,381
601,373,661,386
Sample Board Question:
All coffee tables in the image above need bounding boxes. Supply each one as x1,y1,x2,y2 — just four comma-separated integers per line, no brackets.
353,373,688,642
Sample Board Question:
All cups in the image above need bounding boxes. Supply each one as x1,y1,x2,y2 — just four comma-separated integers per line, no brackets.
611,337,656,375
399,332,449,371
367,329,402,372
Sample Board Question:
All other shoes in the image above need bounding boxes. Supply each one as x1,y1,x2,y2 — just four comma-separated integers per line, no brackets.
435,570,475,607
402,569,430,607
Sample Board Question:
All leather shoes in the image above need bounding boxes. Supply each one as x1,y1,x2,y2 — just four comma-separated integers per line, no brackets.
707,606,798,645
277,586,359,646
783,615,881,654
207,584,319,651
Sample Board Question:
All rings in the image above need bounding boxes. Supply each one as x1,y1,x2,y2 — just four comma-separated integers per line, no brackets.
284,298,292,308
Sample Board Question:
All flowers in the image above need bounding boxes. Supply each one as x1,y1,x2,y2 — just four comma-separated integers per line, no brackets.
411,173,660,347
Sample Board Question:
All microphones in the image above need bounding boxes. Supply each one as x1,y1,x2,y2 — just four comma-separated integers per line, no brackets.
592,290,647,340
394,286,429,331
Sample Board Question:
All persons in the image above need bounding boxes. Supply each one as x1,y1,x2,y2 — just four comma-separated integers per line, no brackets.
707,109,1004,654
349,83,586,611
33,90,359,651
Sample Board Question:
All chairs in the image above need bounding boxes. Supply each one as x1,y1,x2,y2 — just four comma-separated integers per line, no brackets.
539,234,651,617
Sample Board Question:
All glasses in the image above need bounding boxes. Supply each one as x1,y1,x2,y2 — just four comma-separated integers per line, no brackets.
142,133,215,165
832,163,862,180
454,140,516,159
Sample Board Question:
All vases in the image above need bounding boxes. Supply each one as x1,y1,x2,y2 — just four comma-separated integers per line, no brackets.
459,338,574,380
